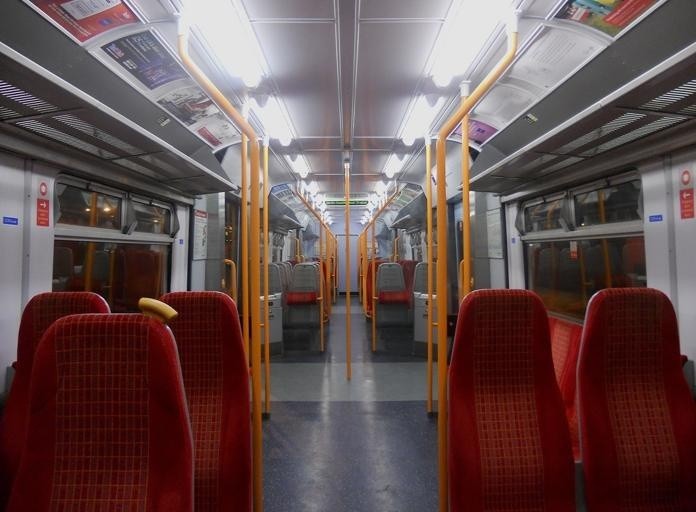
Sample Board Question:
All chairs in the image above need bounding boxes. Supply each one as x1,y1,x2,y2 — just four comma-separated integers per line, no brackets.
159,292,255,511
263,255,330,351
360,260,444,352
576,287,693,512
446,289,572,511
0,291,112,495
9,313,194,512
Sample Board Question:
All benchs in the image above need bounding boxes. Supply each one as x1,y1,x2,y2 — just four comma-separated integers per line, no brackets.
548,314,687,469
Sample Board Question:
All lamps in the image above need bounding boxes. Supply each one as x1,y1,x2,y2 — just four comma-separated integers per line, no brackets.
250,94,446,196
